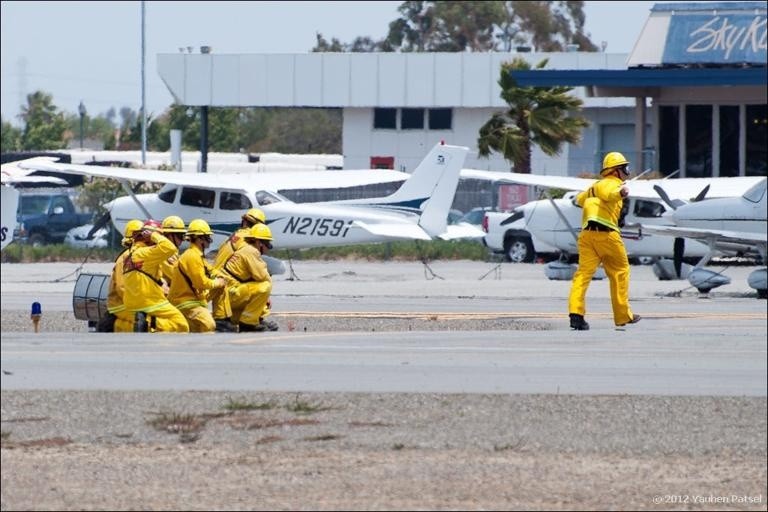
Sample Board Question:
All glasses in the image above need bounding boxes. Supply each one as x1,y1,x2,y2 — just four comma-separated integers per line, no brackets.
615,166,629,175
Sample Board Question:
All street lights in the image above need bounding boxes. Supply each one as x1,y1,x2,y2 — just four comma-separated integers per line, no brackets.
77,102,86,147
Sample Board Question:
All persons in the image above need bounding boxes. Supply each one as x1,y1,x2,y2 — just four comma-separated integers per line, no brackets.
93,208,279,332
569,151,643,330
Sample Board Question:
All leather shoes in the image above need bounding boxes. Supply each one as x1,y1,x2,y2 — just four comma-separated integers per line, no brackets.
570,313,589,330
215,318,278,332
616,314,641,326
134,311,148,332
95,311,118,332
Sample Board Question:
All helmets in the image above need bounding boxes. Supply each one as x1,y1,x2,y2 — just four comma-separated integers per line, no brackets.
603,152,630,170
125,214,213,240
241,207,274,240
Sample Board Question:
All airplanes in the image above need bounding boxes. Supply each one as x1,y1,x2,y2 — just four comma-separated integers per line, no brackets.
457,168,766,263
0,183,19,255
18,141,489,277
1,156,68,184
635,177,768,299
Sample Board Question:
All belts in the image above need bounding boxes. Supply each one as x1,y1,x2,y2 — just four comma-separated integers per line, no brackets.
584,225,614,231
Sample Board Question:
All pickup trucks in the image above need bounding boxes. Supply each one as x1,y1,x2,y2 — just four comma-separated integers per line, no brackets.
14,194,95,248
481,189,578,262
